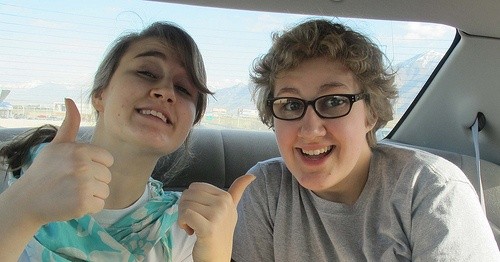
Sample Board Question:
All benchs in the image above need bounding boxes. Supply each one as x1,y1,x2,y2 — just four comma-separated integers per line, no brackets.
0,127,500,255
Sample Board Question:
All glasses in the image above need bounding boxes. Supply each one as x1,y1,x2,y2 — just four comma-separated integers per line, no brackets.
266,88,365,121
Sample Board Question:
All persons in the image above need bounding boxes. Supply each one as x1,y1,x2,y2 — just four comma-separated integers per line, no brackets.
230,19,500,262
0,20,256,262
